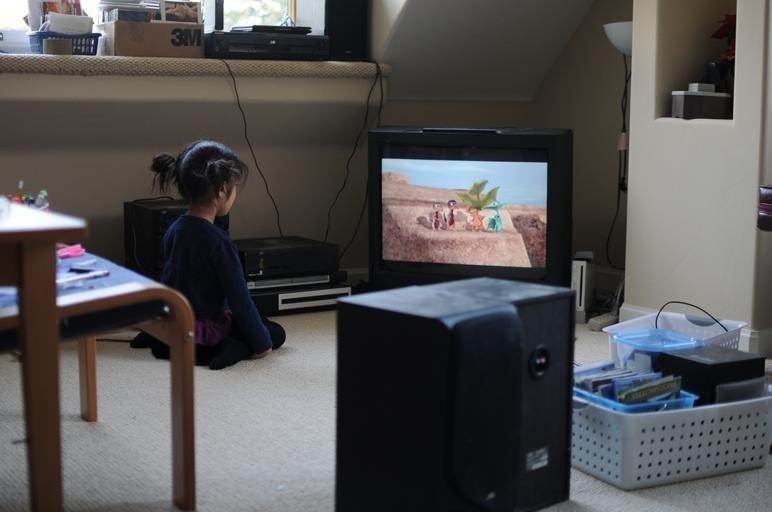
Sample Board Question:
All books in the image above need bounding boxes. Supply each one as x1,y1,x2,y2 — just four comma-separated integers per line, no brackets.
571,363,683,406
22,0,95,57
96,0,205,26
232,26,312,36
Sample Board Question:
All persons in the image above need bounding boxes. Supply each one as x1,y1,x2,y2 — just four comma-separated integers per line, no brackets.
126,139,287,372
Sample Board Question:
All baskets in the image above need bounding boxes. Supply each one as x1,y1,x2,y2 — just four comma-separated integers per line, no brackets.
570,381,772,491
602,312,749,372
572,382,700,413
25,31,102,55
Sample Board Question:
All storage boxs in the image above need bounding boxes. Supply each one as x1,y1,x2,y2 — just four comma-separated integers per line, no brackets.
94,20,205,58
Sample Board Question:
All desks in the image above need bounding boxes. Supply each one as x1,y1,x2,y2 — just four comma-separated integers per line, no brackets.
4,243,197,510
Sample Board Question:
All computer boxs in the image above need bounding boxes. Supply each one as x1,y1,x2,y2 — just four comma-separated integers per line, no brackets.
335,276,579,512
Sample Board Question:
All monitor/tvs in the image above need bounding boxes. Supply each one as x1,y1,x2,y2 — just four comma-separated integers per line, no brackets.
367,123,574,289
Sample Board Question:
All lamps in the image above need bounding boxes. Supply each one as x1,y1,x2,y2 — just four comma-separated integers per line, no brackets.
601,21,632,57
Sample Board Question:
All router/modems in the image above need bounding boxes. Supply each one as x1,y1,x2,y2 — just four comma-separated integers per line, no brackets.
572,261,586,312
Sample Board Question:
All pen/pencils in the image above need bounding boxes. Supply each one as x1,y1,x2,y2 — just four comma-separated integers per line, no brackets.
57,268,109,284
8,180,49,210
